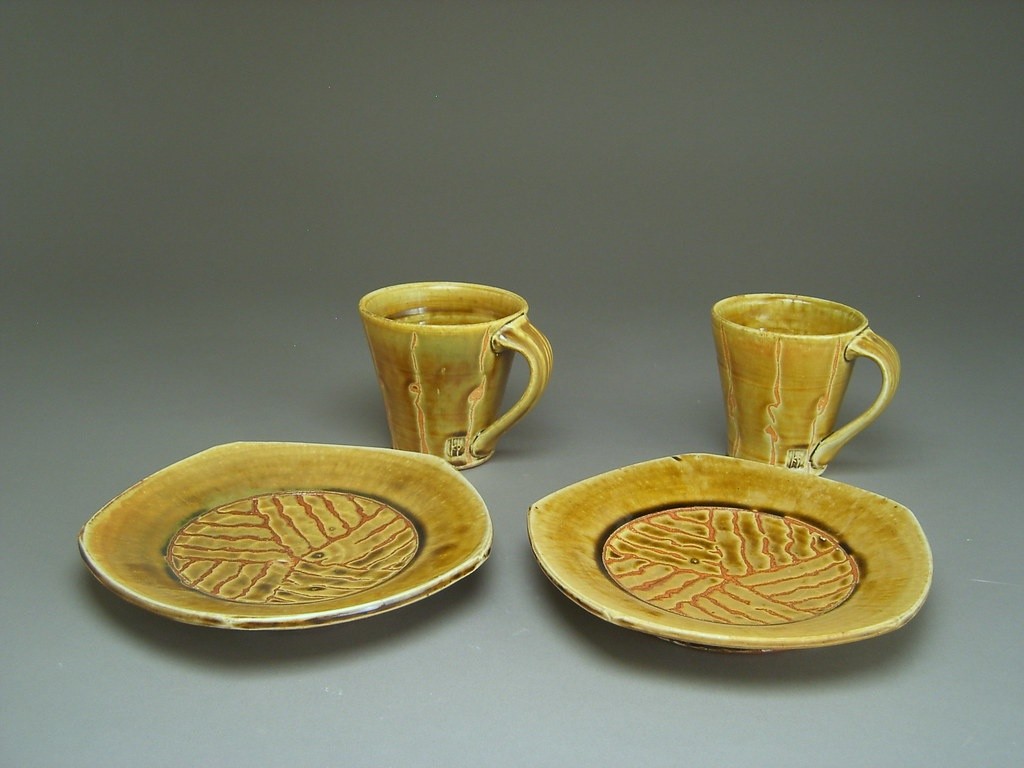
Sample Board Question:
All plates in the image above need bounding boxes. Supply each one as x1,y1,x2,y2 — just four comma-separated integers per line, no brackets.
77,441,494,631
526,453,934,654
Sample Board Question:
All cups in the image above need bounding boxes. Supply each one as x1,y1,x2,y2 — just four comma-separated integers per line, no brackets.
359,281,554,471
710,292,901,476
